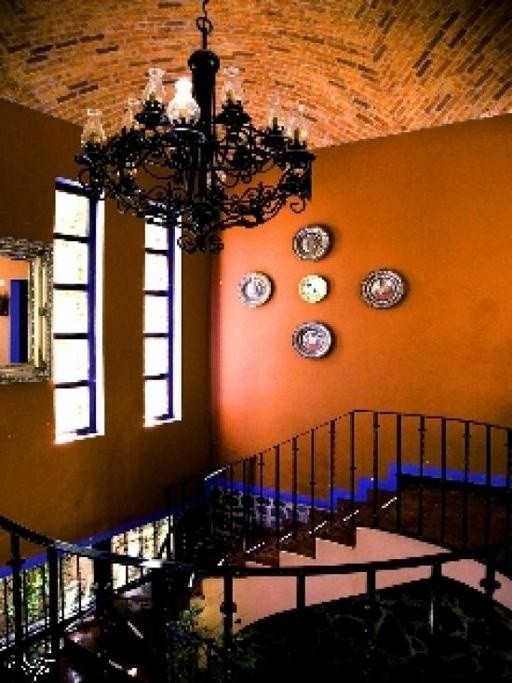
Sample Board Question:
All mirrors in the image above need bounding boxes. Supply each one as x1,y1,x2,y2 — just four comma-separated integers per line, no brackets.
0,236,56,385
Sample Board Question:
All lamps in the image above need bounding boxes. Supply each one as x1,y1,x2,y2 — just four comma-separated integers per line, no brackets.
74,0,318,256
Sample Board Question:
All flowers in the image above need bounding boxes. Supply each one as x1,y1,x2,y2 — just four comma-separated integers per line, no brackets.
165,593,261,682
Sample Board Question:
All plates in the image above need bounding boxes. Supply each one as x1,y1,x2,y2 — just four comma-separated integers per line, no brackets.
292,222,331,262
359,267,407,310
237,270,273,308
291,320,334,358
299,272,329,303
